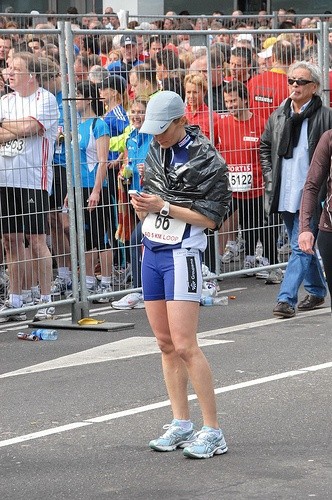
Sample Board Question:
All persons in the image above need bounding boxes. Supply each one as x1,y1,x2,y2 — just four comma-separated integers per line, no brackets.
64,80,115,303
182,71,220,297
124,94,155,309
128,89,237,459
258,60,332,317
298,129,332,314
109,63,162,181
34,56,73,299
214,81,284,284
0,6,62,103
93,75,131,288
0,52,60,322
67,7,332,80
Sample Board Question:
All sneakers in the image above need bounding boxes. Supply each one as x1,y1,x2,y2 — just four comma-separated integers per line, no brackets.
51,275,72,296
114,263,132,285
182,426,229,458
241,259,256,276
148,418,194,452
33,307,56,321
256,258,269,278
0,300,27,323
111,293,146,310
202,278,220,292
264,267,284,284
221,249,240,263
92,284,113,303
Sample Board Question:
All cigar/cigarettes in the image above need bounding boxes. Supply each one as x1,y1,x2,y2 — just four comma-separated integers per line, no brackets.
289,91,295,98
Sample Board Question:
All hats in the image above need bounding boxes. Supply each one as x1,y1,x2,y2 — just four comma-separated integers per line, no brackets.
92,75,124,89
256,45,273,59
237,31,254,47
138,90,184,135
120,34,137,47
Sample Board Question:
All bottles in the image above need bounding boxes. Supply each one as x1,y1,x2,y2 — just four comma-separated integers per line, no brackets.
200,296,229,306
31,329,58,341
122,161,133,186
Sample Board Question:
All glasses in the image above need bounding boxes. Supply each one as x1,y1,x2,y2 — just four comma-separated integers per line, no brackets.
288,79,314,85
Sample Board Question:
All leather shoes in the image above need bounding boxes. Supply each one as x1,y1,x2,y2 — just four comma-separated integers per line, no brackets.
273,302,296,317
298,295,324,310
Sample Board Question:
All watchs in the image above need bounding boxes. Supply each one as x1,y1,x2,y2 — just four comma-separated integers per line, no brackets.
0,118,5,128
160,201,169,218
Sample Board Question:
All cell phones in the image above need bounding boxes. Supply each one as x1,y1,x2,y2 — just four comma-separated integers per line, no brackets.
128,189,142,198
